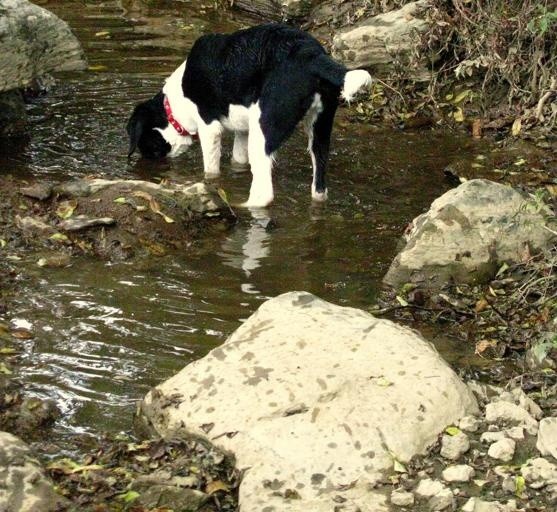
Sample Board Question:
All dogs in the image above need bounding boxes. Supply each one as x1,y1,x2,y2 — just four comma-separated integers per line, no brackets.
125,22,372,209
